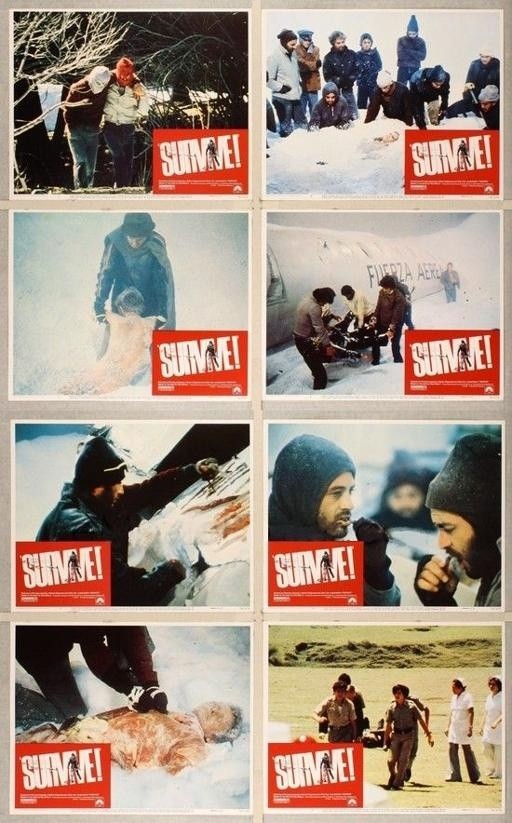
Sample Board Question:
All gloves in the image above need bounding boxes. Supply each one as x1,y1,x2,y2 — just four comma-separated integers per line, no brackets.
200,456,220,481
316,59,321,67
353,518,395,589
280,85,291,94
125,683,168,714
134,83,145,96
94,303,106,321
169,560,186,579
466,82,476,89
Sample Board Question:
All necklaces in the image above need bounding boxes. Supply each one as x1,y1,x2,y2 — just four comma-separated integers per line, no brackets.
444,43,499,118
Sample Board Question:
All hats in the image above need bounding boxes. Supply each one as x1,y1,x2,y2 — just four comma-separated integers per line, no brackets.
425,432,500,517
493,674,502,682
360,33,373,46
299,30,313,38
407,15,418,31
77,437,125,487
380,276,395,290
287,32,297,42
376,69,394,88
457,678,467,687
429,65,446,82
117,288,145,312
122,213,155,236
329,31,343,45
478,85,499,103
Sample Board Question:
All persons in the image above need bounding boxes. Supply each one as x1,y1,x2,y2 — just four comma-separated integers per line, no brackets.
390,273,414,330
310,671,434,791
440,676,485,786
205,340,219,372
319,550,336,582
371,445,439,531
307,80,353,132
36,434,220,605
341,283,374,330
408,63,450,131
363,65,426,130
267,28,310,138
310,311,388,360
413,429,501,606
15,624,169,727
92,213,171,329
321,29,360,120
268,432,401,605
205,138,221,169
68,753,82,784
437,260,461,304
98,54,150,189
465,81,499,131
51,284,169,397
478,673,502,778
68,549,83,582
319,752,335,782
456,139,472,169
395,13,428,86
369,274,405,365
16,699,244,776
61,63,147,186
292,286,336,390
355,32,383,108
456,339,471,371
293,28,322,128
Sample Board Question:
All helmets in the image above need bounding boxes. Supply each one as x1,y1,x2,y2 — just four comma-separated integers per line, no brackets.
116,58,134,86
88,66,111,95
